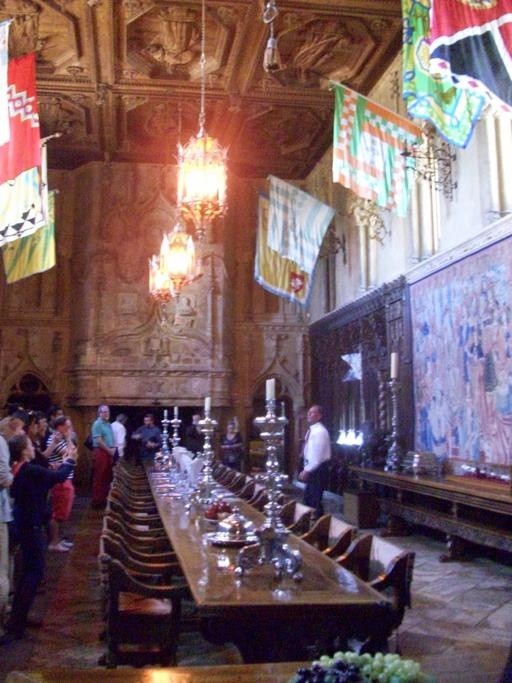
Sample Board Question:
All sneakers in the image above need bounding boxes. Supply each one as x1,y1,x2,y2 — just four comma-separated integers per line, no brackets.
59,539,74,546
47,543,69,552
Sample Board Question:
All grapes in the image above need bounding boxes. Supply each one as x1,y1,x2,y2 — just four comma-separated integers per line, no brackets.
289,650,430,683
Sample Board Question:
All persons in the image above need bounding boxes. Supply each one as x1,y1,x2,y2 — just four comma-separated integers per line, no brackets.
298,405,332,517
107,413,128,462
91,404,116,508
186,414,204,452
220,421,243,471
131,414,161,458
0,401,79,629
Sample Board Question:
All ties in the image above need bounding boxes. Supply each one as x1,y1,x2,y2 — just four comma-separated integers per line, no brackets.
299,430,310,458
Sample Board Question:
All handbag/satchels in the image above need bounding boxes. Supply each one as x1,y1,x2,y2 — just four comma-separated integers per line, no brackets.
84,434,94,451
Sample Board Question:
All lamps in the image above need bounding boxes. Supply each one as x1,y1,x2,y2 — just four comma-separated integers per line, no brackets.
175,0,231,238
148,252,178,313
160,220,200,291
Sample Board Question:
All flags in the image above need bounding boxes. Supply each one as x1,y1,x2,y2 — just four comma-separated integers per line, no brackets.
253,188,319,308
1,20,57,285
327,78,429,215
428,0,512,119
399,0,485,149
267,174,336,273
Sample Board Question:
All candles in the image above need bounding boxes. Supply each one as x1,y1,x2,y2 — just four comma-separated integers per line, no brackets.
163,408,169,419
266,377,274,401
174,405,179,420
204,396,211,412
391,351,398,379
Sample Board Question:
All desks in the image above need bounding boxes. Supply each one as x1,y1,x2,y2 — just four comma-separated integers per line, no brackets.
348,466,512,563
2,658,320,683
